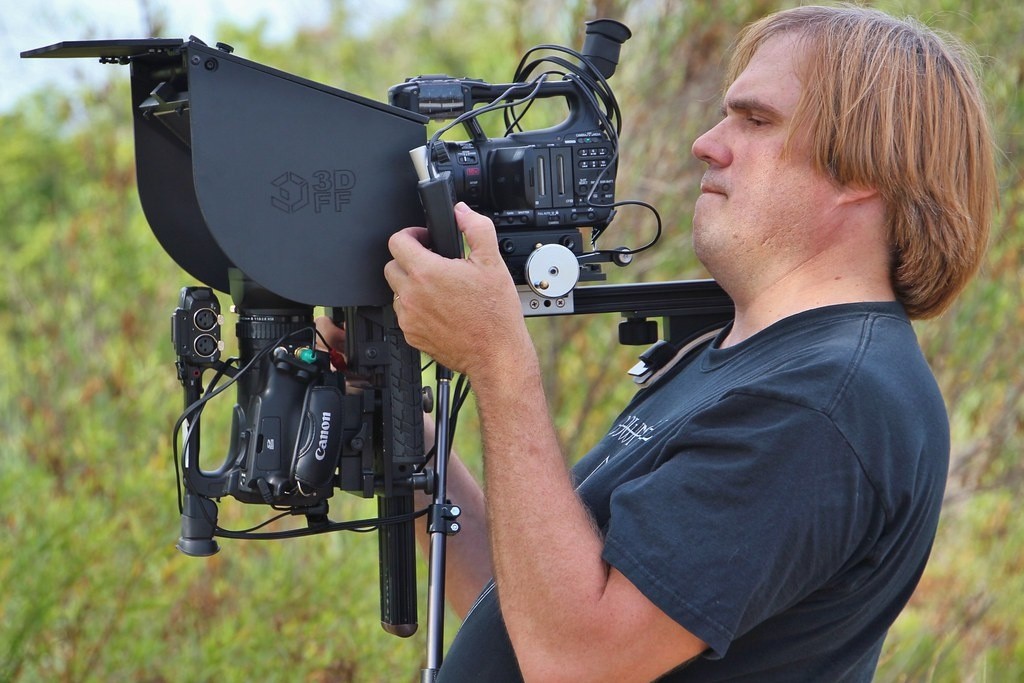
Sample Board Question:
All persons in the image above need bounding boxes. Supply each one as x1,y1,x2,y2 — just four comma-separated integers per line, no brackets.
313,6,999,683
